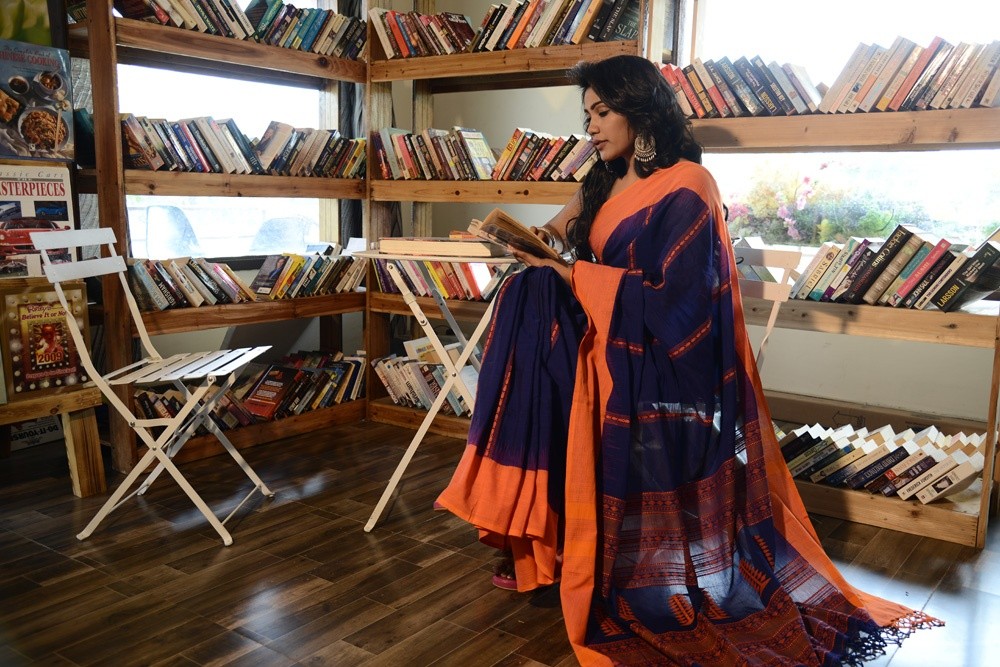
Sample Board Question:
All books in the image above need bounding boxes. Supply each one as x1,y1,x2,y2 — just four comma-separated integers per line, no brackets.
788,224,1000,315
449,230,479,238
248,238,367,299
68,0,255,41
254,121,366,179
73,108,264,176
469,0,647,54
133,349,366,444
467,207,567,265
654,54,822,118
375,126,497,180
245,0,369,63
491,126,598,182
733,236,793,288
370,336,482,418
818,36,1000,113
126,256,256,311
0,40,93,394
368,6,476,59
775,423,999,505
378,236,506,259
371,242,513,303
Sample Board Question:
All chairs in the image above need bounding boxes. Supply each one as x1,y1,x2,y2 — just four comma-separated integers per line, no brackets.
27,225,280,548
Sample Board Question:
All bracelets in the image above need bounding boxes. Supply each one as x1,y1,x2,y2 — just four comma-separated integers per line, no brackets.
536,227,556,249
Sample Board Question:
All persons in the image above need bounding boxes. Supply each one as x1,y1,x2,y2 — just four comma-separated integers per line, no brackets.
432,55,947,667
265,258,286,288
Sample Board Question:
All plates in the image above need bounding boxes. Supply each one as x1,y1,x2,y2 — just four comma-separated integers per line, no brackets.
33,72,69,101
0,88,26,127
17,106,70,153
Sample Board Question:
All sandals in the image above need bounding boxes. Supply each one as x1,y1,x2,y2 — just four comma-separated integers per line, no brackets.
492,553,563,593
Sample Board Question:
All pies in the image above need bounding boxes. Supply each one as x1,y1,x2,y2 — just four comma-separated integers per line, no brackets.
0,89,20,122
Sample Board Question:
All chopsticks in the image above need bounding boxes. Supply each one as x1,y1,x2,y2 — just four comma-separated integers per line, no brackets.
55,109,62,154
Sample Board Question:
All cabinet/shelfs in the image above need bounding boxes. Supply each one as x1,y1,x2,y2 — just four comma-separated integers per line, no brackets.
370,0,650,443
621,102,1000,552
55,0,365,479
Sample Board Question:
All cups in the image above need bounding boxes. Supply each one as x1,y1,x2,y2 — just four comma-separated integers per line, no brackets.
39,70,63,94
8,75,29,95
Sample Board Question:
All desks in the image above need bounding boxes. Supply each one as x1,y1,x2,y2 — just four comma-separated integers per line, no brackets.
348,249,536,535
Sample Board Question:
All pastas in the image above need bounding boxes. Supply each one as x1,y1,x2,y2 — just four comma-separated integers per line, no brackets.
22,111,66,149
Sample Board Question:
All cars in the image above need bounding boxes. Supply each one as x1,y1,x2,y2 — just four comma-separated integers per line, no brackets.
129,201,320,262
